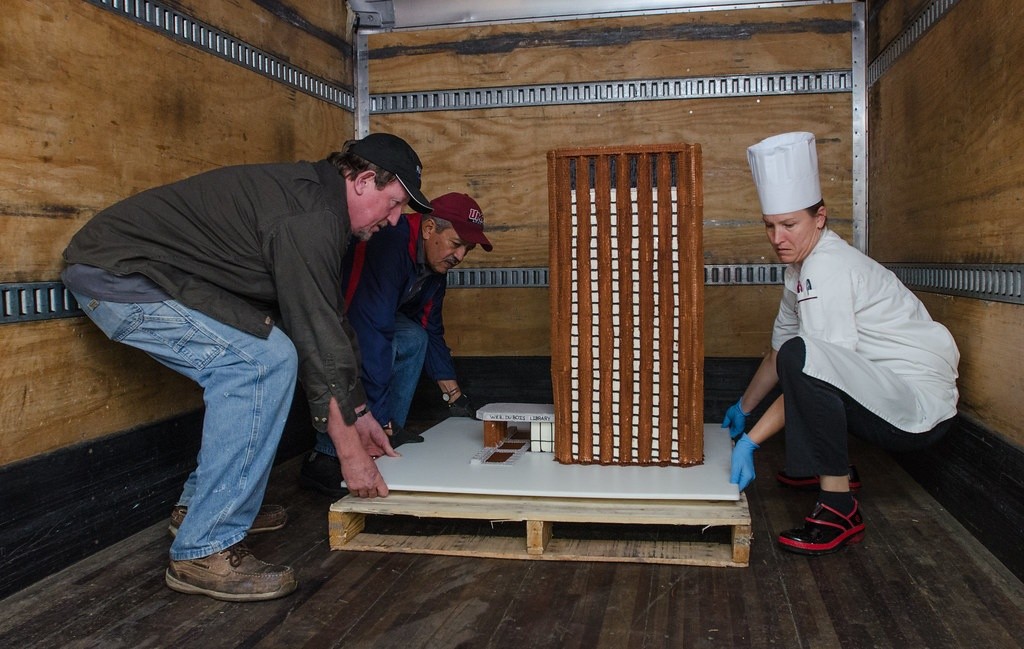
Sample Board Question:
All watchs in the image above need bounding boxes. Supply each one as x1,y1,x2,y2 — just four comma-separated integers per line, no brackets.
443,388,459,401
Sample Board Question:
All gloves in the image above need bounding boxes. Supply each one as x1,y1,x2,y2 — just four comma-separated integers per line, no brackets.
730,432,760,493
387,421,424,449
721,396,750,438
448,394,481,421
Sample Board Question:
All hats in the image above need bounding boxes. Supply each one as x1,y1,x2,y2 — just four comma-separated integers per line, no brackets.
431,192,494,251
747,131,822,215
347,133,434,214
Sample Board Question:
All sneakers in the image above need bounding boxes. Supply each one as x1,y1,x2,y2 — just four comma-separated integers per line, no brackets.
777,465,863,489
301,450,350,497
164,541,298,601
778,497,865,556
168,504,288,537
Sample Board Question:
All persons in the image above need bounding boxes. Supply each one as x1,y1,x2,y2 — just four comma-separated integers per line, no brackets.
722,132,961,553
59,132,434,602
305,191,493,500
